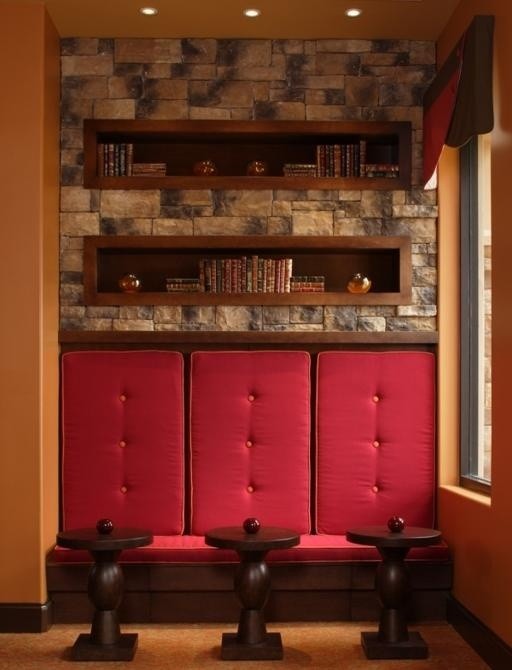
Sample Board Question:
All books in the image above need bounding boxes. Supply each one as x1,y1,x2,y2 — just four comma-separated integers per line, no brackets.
98,141,167,177
282,140,401,179
165,255,325,294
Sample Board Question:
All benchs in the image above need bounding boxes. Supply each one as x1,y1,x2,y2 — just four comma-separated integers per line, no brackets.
46,331,452,623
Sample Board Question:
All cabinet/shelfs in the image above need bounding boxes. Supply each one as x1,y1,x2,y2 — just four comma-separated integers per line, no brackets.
84,119,412,306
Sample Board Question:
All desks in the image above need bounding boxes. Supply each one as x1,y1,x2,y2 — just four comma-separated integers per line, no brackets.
57,520,152,661
346,517,441,659
205,519,300,660
